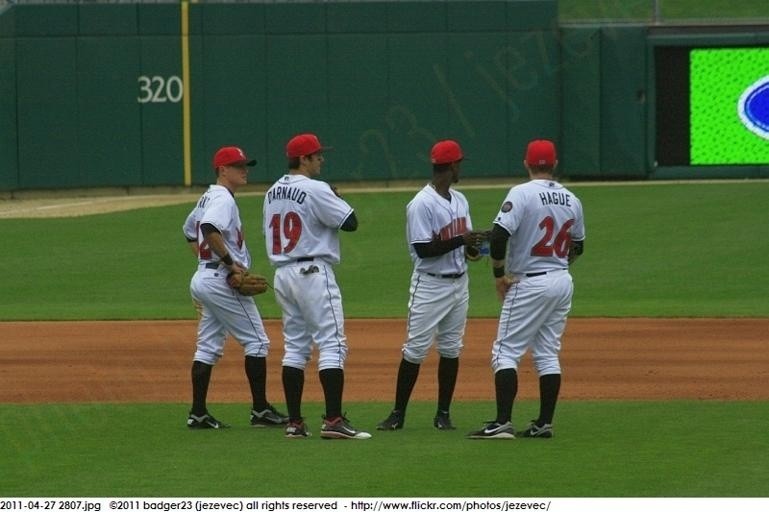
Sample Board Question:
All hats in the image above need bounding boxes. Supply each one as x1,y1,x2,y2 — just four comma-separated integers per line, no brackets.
211,145,257,169
430,140,471,165
526,138,556,167
285,133,334,162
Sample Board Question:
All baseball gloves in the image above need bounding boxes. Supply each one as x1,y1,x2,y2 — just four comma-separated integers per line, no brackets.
230,274,267,295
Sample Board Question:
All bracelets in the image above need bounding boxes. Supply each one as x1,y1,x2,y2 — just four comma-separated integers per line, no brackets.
221,252,235,268
492,263,507,277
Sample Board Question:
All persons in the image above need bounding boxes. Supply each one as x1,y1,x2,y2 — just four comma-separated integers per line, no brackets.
260,132,373,441
181,144,290,432
376,140,489,432
465,139,589,440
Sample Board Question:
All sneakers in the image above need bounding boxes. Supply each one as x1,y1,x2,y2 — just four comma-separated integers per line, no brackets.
187,412,231,429
465,420,515,439
284,418,312,438
515,423,553,439
249,403,290,427
320,417,372,440
432,409,456,432
375,409,405,429
